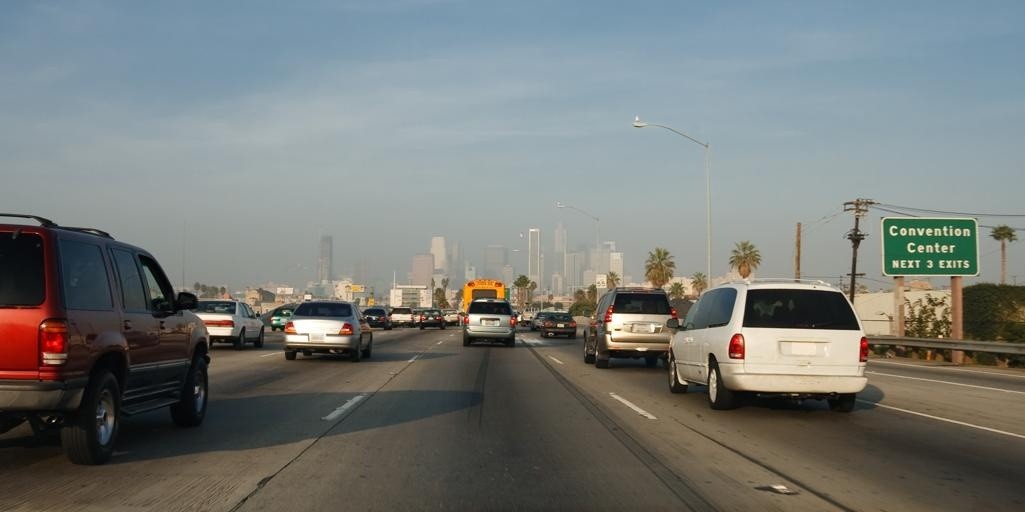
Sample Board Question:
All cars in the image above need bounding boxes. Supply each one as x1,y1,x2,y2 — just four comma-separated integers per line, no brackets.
189,295,267,352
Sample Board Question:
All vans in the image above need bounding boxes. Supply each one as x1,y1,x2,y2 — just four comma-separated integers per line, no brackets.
665,276,871,415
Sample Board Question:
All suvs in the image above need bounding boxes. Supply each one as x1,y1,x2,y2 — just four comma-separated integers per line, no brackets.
1,212,217,463
583,286,680,372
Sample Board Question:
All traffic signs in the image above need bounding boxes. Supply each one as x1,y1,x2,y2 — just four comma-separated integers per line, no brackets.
878,215,982,277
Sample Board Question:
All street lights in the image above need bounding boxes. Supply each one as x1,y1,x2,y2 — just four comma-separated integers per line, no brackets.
633,115,712,294
554,201,602,303
